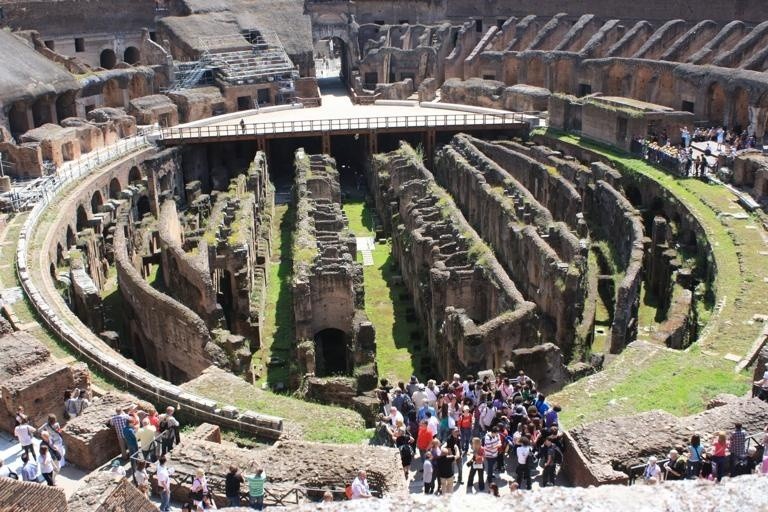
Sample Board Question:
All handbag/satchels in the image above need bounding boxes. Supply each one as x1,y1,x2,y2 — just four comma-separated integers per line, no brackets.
63,410,70,419
503,436,515,452
160,421,168,433
526,452,538,468
473,460,483,469
402,445,414,460
188,489,203,501
137,484,148,493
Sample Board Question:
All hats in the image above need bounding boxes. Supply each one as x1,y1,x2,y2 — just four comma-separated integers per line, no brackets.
410,375,418,383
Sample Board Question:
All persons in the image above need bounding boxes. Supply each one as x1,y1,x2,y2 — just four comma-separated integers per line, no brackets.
636,123,757,178
753,363,768,400
0,386,94,487
108,401,266,512
641,421,768,484
377,371,563,493
318,470,373,504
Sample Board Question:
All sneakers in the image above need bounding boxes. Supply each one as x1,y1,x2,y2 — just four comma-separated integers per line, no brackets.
53,449,62,460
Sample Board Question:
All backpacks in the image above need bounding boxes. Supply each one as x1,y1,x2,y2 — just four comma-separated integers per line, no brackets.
550,443,564,464
